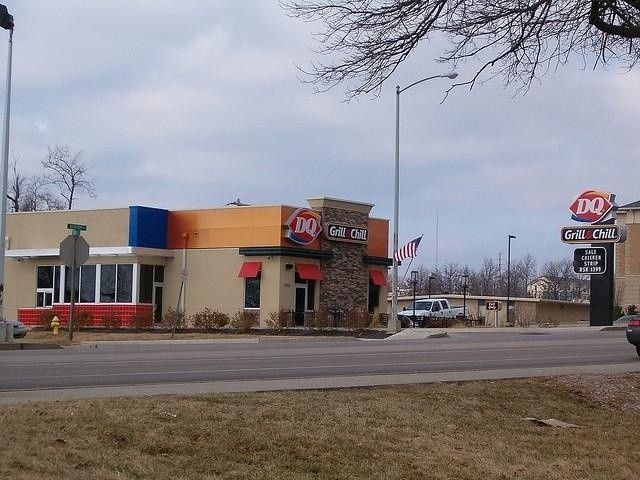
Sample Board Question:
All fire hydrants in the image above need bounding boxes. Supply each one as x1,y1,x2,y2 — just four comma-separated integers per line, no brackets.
50,316,60,335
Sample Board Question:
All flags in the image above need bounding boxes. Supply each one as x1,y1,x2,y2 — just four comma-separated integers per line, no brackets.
394,236,422,263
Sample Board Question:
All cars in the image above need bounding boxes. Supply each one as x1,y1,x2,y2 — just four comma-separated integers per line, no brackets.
626,315,640,356
617,315,640,321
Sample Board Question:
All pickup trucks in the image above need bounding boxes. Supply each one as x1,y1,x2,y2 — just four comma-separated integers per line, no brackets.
397,298,469,326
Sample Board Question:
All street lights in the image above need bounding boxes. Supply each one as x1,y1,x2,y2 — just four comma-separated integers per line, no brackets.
411,270,418,328
461,275,469,317
388,73,459,335
